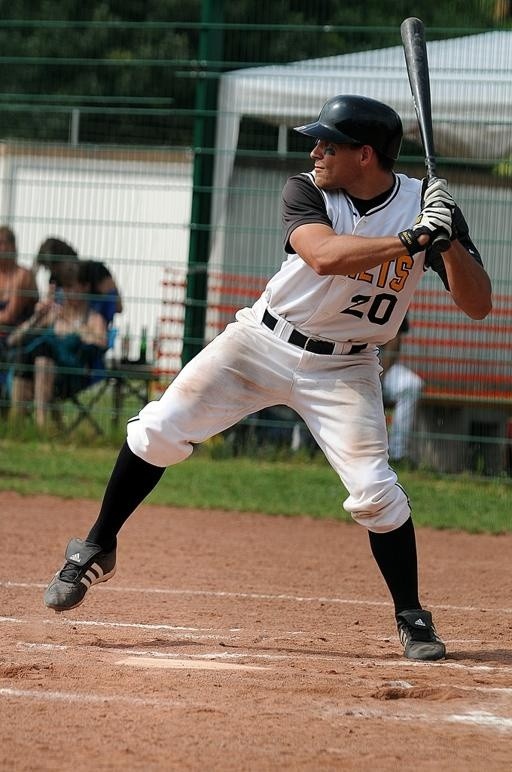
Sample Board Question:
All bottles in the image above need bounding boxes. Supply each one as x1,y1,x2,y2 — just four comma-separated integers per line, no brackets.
119,321,147,363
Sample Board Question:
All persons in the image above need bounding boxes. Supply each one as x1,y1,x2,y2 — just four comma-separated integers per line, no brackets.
380,311,424,471
45,95,491,659
0,227,122,430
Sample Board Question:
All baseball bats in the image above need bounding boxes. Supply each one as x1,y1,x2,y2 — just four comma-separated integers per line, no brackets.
401,18,449,255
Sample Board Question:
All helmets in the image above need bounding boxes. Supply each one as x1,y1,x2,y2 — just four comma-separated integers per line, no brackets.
291,94,404,161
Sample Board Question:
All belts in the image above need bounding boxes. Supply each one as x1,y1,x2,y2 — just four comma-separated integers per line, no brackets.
262,309,369,355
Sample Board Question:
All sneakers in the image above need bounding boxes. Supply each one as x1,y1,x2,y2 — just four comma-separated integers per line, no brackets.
395,609,447,662
44,538,117,611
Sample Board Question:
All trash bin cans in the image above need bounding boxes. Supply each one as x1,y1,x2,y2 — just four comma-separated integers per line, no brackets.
411,405,470,474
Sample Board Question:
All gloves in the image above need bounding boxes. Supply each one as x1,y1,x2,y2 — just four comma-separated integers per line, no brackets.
397,205,453,257
421,176,459,242
